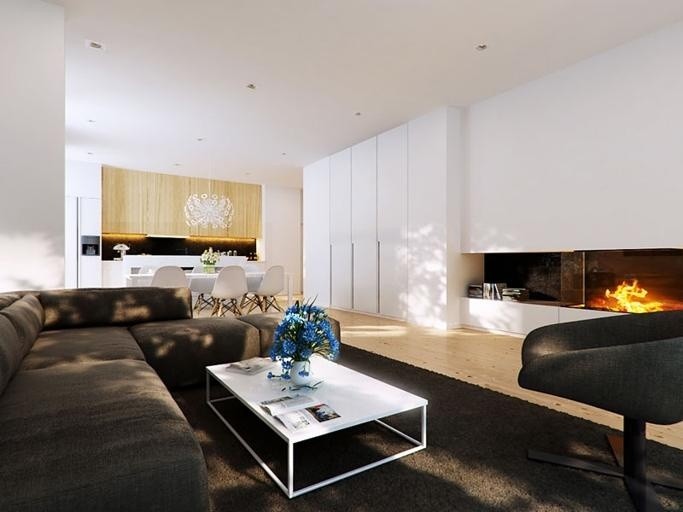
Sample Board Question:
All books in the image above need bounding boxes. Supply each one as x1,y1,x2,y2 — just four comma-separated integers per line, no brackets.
468,283,529,302
225,356,275,376
259,395,341,434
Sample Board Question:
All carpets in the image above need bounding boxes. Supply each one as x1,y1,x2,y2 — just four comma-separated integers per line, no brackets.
169,343,683,512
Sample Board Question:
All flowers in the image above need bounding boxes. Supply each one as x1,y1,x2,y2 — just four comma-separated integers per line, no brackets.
267,295,340,380
200,249,222,262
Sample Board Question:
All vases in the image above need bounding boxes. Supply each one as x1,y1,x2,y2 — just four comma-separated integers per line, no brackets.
204,264,216,274
291,361,310,386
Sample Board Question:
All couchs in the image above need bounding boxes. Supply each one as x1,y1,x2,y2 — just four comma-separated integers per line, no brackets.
1,287,341,512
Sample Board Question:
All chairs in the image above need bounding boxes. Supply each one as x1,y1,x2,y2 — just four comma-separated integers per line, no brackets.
209,265,248,318
246,264,286,315
151,265,188,287
518,309,683,512
